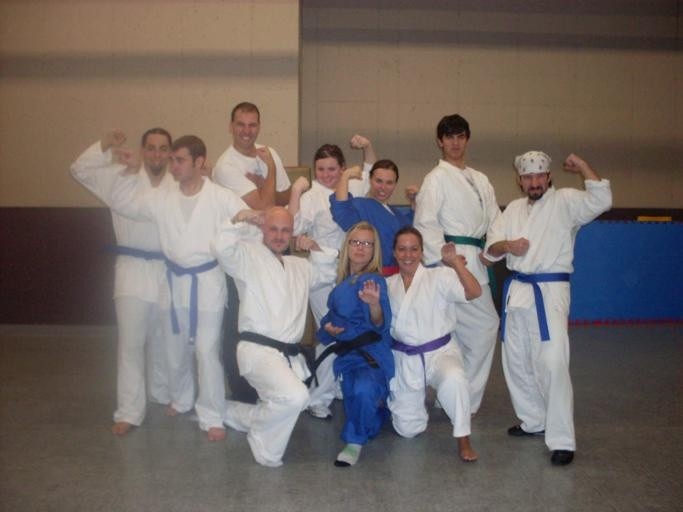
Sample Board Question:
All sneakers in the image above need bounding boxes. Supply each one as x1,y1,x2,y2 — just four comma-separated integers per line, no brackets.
309,404,332,420
551,450,574,464
508,425,544,436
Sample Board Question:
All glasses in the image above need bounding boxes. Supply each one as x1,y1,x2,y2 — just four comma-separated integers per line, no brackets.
348,239,374,248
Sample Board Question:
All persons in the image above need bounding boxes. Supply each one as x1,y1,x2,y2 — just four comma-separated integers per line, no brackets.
484,149,614,465
210,100,292,402
316,221,393,469
412,114,502,420
106,133,253,443
381,225,482,463
211,206,338,469
286,131,377,422
328,159,420,276
70,129,172,438
